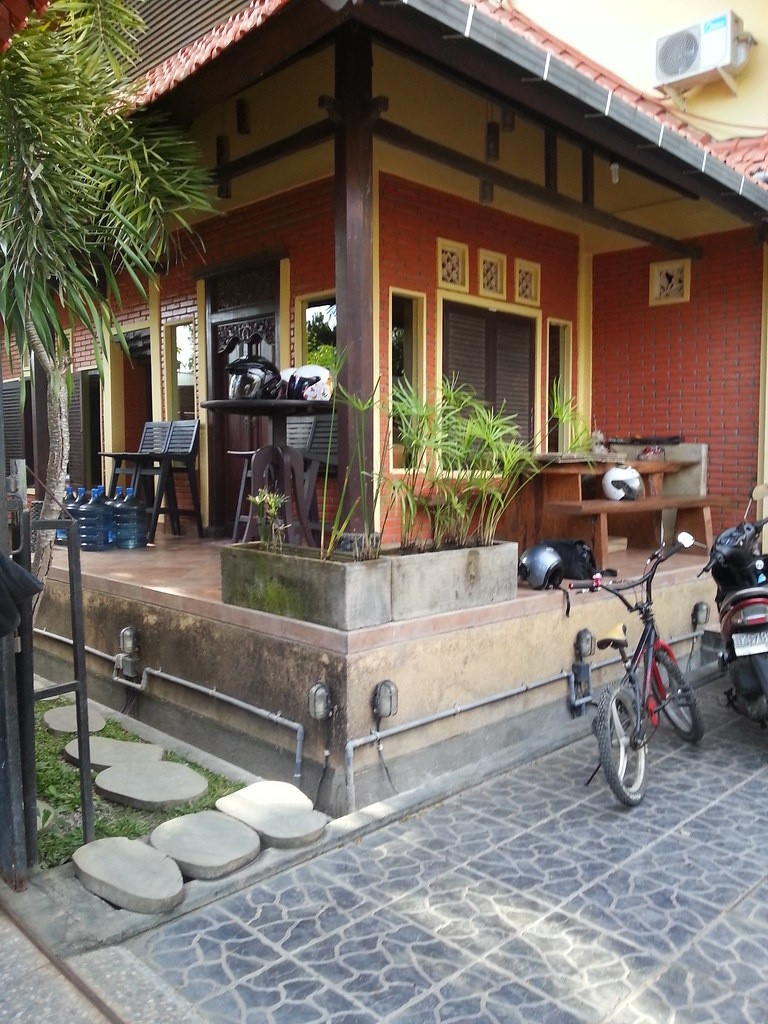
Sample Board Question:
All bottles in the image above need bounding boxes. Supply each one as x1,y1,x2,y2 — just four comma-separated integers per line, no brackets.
78,489,106,551
98,485,113,545
70,489,88,507
105,488,123,547
116,487,146,549
55,487,76,543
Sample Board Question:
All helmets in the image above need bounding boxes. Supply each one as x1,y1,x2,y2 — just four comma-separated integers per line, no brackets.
224,355,333,402
519,546,563,589
602,465,646,501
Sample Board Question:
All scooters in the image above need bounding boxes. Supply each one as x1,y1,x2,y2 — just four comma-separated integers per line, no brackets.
676,483,768,728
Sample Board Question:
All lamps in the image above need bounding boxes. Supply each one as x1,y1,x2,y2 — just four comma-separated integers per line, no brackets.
574,628,596,656
690,601,710,624
371,679,398,718
118,626,139,655
308,681,332,720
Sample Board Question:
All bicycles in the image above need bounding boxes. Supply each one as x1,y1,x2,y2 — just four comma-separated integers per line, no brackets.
568,541,705,806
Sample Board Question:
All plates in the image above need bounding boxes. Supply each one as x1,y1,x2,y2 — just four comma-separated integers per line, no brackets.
278,367,297,380
291,365,333,399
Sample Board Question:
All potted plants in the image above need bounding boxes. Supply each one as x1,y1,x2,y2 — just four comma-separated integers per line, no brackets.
220,342,393,632
380,369,604,622
290,345,337,402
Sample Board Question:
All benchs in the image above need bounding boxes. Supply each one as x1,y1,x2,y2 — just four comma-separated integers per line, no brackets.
547,496,732,572
411,478,507,540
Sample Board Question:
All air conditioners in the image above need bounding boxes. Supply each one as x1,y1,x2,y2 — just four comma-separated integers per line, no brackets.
653,9,744,99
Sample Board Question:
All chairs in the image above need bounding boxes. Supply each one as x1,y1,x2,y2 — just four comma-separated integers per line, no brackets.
97,422,176,537
283,416,337,549
124,420,204,544
226,416,316,545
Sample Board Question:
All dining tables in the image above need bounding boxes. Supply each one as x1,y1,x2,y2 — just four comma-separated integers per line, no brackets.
200,399,338,549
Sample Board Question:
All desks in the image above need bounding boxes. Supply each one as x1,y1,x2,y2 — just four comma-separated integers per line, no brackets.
506,458,699,568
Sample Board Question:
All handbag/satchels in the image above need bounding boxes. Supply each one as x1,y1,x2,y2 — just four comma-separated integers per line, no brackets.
544,539,598,579
